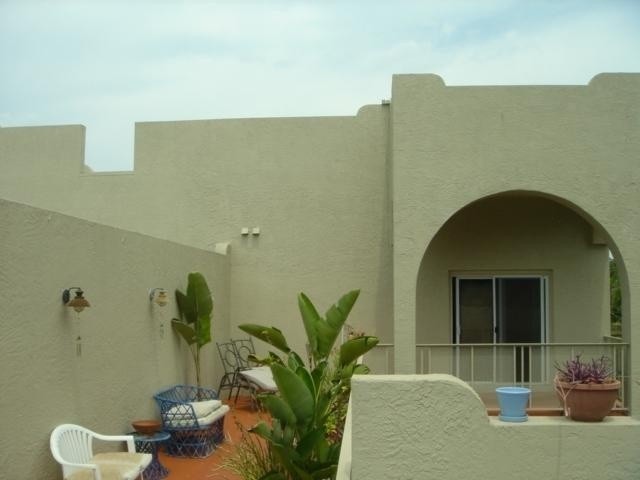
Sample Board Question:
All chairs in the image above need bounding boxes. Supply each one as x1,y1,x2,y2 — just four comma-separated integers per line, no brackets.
215,336,278,412
50,384,229,479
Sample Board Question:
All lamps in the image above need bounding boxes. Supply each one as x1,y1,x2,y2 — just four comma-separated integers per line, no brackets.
62,286,92,314
149,286,168,307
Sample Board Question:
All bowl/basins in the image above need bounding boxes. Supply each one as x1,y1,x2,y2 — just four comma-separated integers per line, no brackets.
131,420,162,437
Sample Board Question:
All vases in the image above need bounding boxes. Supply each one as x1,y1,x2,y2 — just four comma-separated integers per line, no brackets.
553,375,621,422
495,386,532,419
